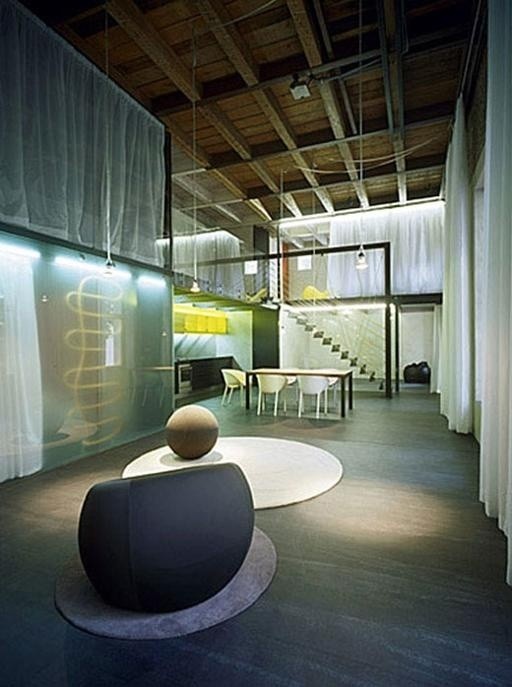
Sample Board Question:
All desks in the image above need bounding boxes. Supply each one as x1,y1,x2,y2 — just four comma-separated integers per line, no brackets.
246,368,352,418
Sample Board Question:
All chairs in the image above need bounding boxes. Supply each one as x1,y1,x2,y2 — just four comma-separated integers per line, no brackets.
256,374,288,416
320,368,339,408
297,375,329,419
221,369,252,407
285,375,299,404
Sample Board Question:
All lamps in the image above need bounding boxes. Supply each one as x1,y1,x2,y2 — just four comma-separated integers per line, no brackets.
104,259,116,275
280,324,287,335
356,244,368,270
190,282,200,293
311,324,318,337
